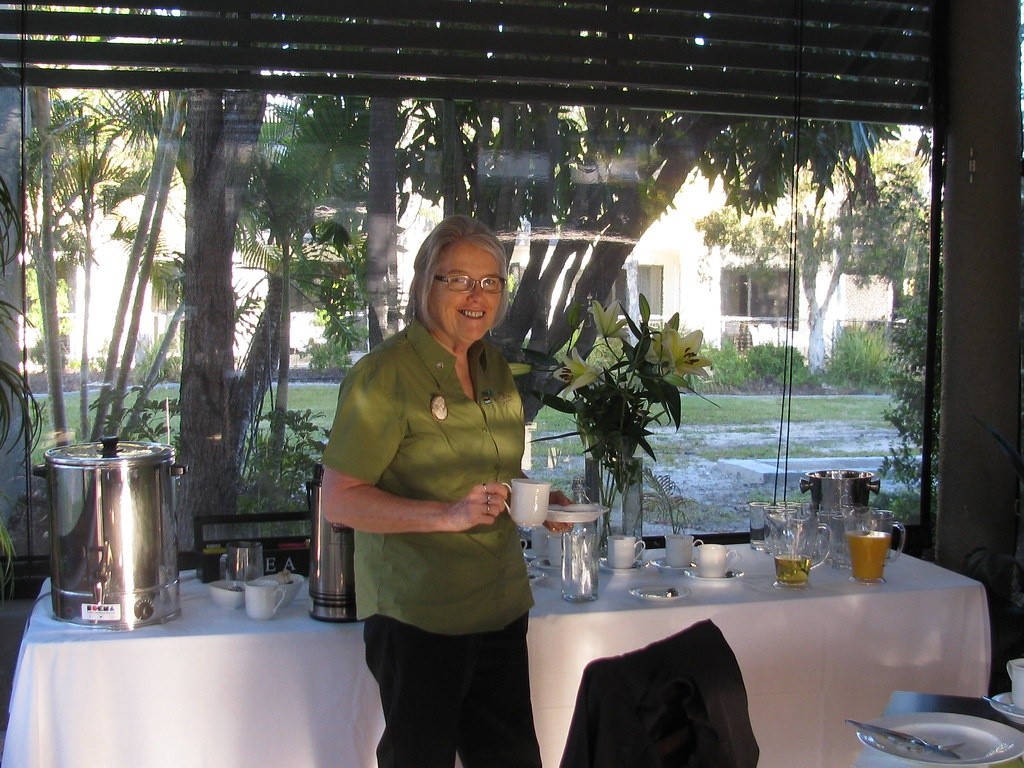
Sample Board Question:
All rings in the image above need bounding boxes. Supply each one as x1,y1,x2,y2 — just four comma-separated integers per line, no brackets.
483,482,492,514
552,527,557,531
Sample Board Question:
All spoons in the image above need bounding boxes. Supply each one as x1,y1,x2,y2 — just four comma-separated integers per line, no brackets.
981,695,1024,715
846,718,965,751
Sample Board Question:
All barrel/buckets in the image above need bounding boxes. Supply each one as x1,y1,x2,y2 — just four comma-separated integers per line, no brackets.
32,434,190,634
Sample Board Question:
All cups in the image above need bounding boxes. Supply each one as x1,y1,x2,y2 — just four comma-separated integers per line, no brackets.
548,534,562,567
606,535,646,569
502,478,552,527
1006,658,1024,715
697,543,738,578
664,534,704,567
245,578,284,620
219,541,263,582
531,527,549,557
560,530,599,602
749,499,906,592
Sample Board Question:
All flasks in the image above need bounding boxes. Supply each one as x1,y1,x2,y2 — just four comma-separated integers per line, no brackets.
306,461,355,623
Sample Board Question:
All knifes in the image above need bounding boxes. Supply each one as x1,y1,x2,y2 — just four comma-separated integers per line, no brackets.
845,719,961,760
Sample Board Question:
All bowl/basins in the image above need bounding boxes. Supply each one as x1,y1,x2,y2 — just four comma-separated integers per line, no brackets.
208,579,246,609
254,574,305,609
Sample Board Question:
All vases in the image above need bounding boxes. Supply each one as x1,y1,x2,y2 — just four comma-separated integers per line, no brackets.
585,456,643,566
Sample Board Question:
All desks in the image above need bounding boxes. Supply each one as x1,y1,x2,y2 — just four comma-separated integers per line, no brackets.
0,543,991,768
853,691,1024,768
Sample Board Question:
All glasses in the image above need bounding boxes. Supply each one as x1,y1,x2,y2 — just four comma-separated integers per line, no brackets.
434,274,508,293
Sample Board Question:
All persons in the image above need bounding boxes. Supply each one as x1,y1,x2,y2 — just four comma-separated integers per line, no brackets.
319,215,575,768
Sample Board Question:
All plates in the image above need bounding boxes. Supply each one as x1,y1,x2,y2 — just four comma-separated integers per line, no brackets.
684,568,744,580
598,558,649,575
531,558,561,576
628,584,690,602
547,502,610,523
649,557,698,572
855,711,1024,768
523,548,537,561
989,692,1024,725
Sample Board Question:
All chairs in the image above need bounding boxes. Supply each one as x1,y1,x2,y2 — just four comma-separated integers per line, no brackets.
559,619,759,768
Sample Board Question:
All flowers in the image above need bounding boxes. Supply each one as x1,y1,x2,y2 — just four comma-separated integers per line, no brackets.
506,293,722,547
